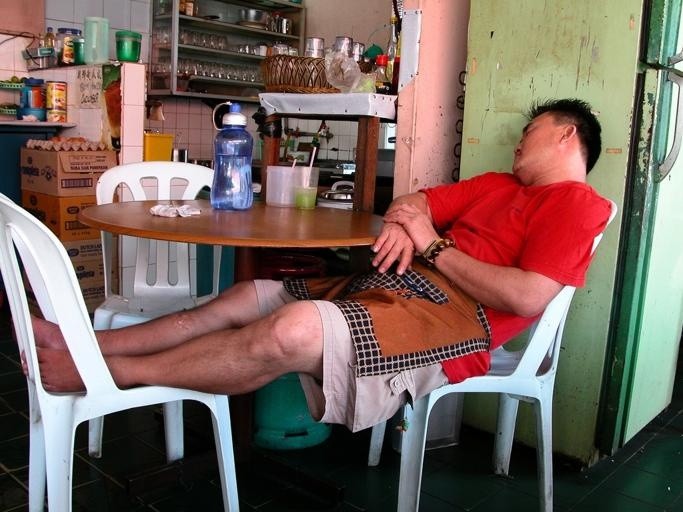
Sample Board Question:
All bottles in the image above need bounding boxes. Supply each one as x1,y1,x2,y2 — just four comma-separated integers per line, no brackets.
45,27,82,65
211,99,253,211
370,54,391,94
159,0,199,16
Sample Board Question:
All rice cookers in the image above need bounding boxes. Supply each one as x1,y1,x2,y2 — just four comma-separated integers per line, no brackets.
320,179,354,209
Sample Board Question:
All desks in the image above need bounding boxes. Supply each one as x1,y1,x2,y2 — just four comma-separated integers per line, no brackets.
255,89,398,213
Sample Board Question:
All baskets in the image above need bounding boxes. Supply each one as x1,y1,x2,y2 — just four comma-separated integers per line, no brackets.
264,54,372,93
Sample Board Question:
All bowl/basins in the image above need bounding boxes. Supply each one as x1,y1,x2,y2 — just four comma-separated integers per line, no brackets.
238,7,268,25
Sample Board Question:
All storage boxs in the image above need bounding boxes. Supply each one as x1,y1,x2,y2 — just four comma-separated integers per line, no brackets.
17,146,118,198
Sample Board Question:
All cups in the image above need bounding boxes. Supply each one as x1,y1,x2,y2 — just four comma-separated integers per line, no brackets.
269,37,365,85
295,186,317,209
155,28,268,81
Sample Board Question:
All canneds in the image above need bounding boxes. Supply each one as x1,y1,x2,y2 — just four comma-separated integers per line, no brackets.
46,81,65,109
46,111,66,121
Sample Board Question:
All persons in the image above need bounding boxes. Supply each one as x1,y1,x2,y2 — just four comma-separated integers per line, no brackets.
11,96,615,434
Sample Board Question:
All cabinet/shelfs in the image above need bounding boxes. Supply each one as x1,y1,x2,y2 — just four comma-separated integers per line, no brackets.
147,0,307,106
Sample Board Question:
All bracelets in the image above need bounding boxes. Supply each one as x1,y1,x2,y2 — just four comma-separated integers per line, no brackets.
424,234,454,266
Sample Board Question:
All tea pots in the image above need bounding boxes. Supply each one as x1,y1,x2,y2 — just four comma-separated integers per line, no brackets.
82,17,110,64
